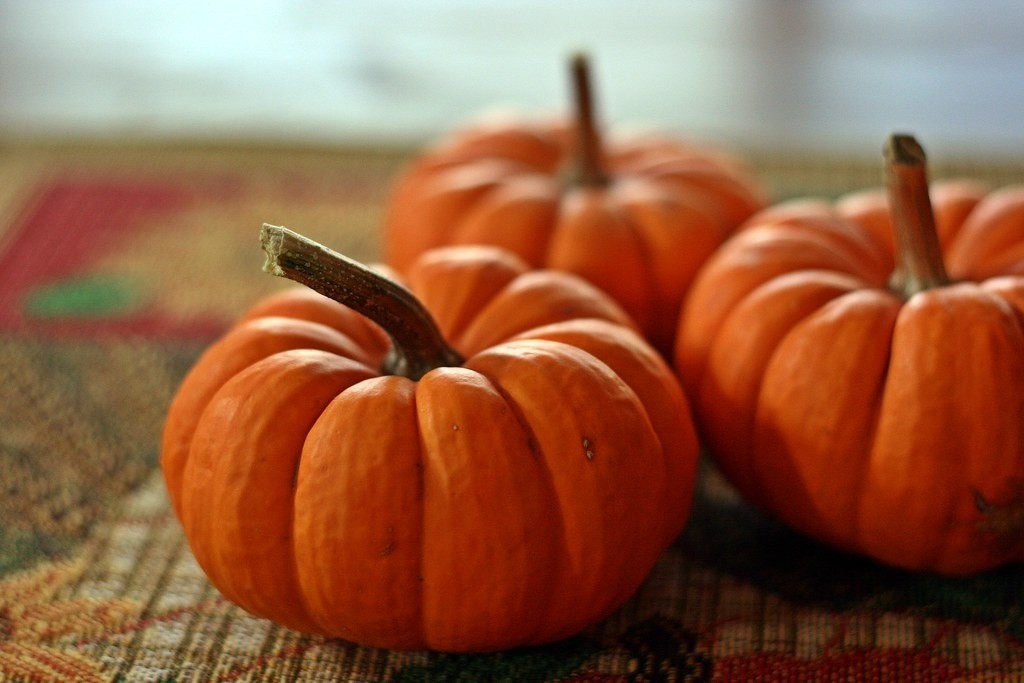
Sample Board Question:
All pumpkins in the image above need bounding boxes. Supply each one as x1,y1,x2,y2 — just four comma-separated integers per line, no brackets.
673,130,1024,574
377,55,768,363
160,221,701,650
